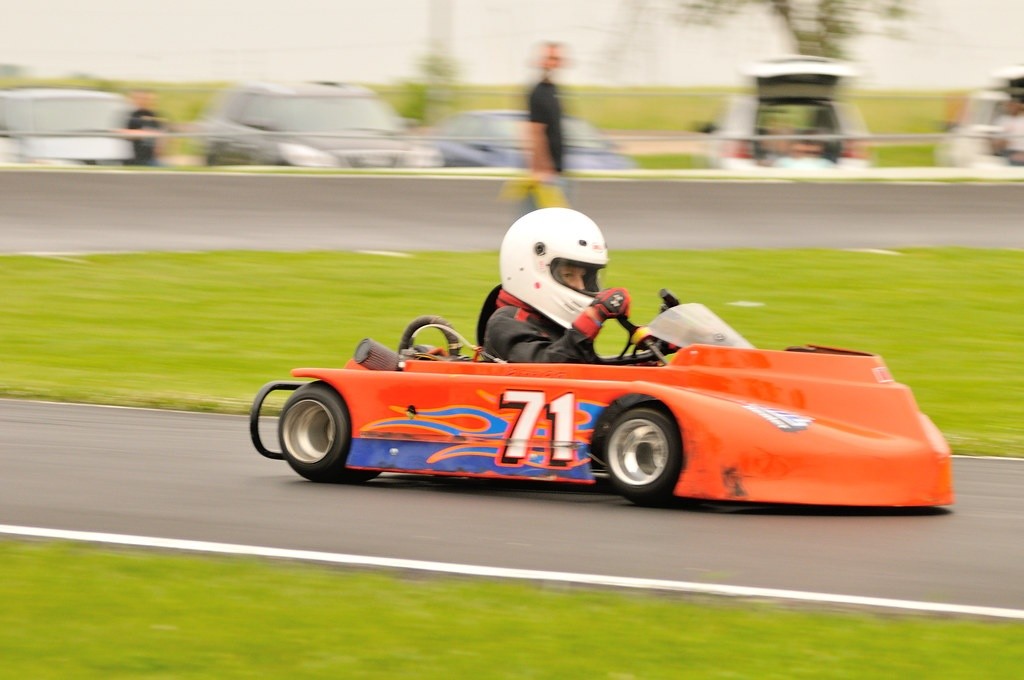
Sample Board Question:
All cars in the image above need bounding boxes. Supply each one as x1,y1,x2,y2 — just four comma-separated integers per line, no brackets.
195,82,436,169
1,84,148,166
439,111,639,175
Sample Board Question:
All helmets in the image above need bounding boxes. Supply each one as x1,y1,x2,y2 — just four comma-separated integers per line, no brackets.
500,208,608,330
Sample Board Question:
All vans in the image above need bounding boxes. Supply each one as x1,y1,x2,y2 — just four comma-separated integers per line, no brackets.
698,57,875,173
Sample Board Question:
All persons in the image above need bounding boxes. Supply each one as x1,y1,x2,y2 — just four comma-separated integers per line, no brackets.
528,42,564,171
483,208,680,366
128,93,161,166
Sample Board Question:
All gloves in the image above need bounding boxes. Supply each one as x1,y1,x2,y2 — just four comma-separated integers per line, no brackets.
572,288,631,340
659,340,679,354
